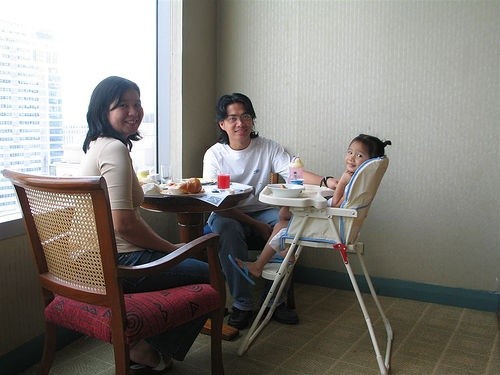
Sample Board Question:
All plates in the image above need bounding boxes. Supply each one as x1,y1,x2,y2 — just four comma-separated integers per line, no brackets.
180,181,216,185
160,190,205,197
160,183,180,190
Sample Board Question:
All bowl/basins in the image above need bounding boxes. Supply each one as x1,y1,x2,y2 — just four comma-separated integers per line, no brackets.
268,184,304,198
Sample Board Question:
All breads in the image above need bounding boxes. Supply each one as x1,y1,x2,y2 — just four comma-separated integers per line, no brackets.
178,177,202,193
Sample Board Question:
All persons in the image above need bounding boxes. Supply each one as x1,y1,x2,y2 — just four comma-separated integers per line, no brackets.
203,92,299,329
228,133,392,279
69,76,210,375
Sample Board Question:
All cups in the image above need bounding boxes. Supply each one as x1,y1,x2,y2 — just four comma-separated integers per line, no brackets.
168,187,184,195
161,161,173,184
218,173,230,190
290,179,303,193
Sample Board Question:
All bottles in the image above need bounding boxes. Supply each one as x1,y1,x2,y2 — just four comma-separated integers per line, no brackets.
288,156,304,184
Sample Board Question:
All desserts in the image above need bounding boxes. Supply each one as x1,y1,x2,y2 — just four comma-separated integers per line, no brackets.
168,185,186,195
140,183,161,195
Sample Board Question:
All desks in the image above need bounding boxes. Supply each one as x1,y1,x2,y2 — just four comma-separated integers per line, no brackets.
140,179,254,342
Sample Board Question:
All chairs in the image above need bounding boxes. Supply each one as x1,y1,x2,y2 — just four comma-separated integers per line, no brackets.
1,169,227,375
237,157,393,375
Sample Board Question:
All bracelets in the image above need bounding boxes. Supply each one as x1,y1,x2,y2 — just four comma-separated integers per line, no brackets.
320,176,334,188
